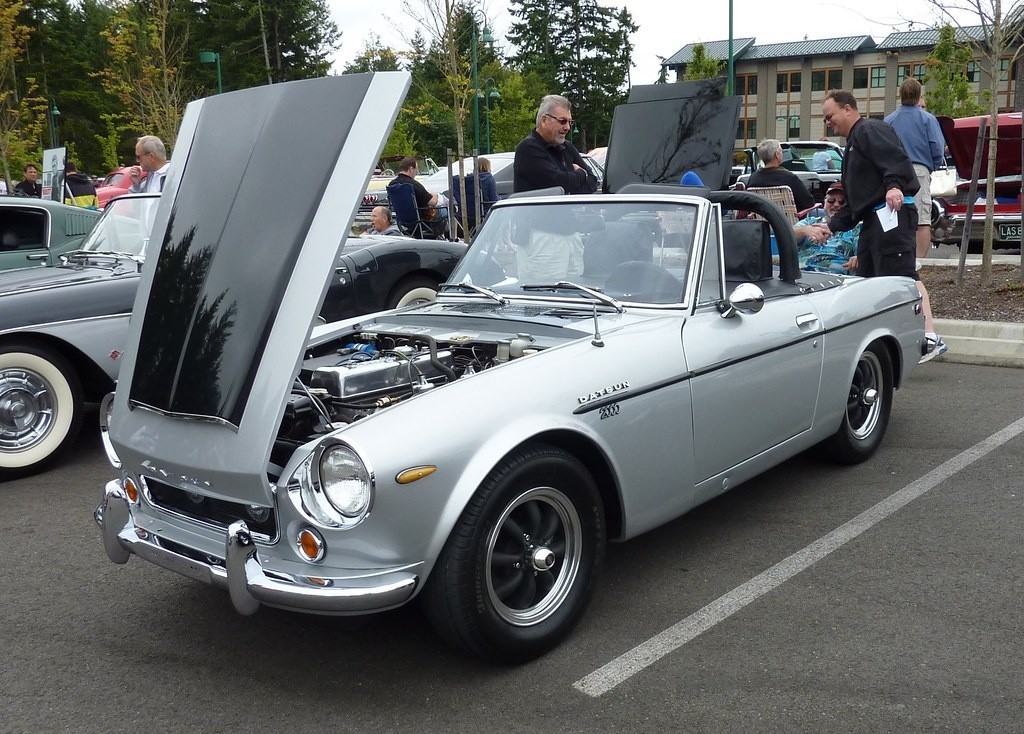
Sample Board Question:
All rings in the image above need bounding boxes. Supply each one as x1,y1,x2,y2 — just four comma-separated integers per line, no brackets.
897,200,901,203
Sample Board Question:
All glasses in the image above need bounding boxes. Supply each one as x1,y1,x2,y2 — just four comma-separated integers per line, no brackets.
822,107,846,123
546,113,574,126
826,198,845,204
136,151,151,160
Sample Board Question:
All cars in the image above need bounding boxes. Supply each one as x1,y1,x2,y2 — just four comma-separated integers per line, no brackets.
928,110,1023,255
0,68,948,673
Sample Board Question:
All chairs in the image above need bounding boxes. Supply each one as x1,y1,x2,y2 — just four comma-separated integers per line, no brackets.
656,210,697,269
578,225,652,295
747,187,822,255
385,175,493,244
704,218,774,298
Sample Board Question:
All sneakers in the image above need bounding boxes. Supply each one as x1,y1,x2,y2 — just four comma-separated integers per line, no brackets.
917,335,948,364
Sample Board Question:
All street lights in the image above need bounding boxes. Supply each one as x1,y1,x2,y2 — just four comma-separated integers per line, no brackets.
484,75,500,153
472,9,494,155
34,94,61,149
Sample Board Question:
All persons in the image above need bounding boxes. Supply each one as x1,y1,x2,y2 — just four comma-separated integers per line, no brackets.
388,157,460,242
51,154,66,203
511,95,598,282
916,95,949,170
813,137,835,171
64,162,99,208
360,206,405,237
809,91,948,365
90,175,101,188
883,78,947,271
127,135,171,257
791,182,863,277
14,164,42,199
746,139,815,221
467,158,516,253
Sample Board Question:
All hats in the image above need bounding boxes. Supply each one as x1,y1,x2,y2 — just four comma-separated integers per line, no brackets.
825,182,844,192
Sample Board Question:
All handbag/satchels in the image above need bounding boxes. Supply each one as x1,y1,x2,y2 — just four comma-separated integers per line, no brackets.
929,156,958,196
